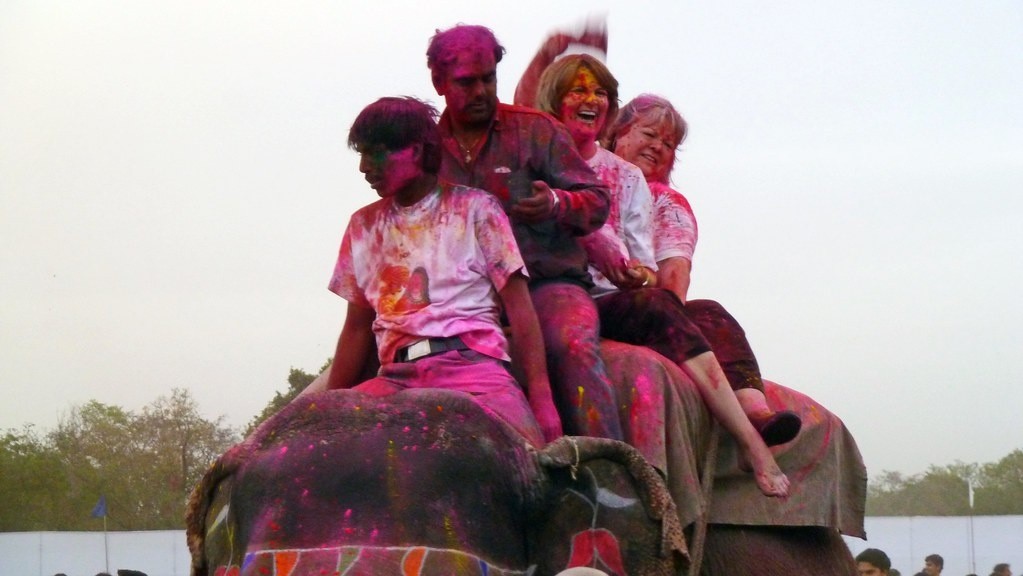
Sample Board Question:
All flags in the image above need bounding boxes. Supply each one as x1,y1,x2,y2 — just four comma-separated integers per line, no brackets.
90,497,107,520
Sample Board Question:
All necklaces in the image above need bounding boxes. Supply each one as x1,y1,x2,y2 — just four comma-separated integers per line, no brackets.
459,130,482,163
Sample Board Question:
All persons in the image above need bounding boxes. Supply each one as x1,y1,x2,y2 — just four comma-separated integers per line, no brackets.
292,14,792,497
989,563,1012,576
856,548,891,576
914,554,943,576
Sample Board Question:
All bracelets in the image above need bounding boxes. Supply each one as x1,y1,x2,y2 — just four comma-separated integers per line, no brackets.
643,273,654,287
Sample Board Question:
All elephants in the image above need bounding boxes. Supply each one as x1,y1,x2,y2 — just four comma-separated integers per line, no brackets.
185,339,863,576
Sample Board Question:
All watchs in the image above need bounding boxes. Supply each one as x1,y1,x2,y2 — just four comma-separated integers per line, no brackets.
550,189,560,216
